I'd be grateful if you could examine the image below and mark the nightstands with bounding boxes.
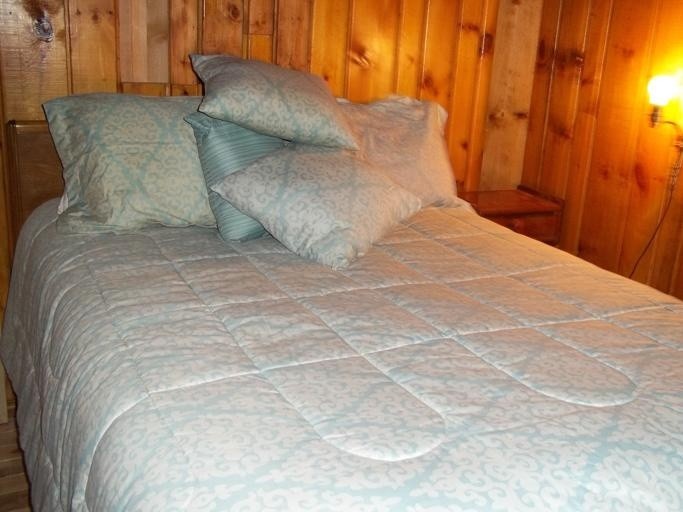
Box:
[457,184,566,250]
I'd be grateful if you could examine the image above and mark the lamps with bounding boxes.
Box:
[647,75,679,130]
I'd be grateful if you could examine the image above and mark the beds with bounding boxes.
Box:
[0,118,682,512]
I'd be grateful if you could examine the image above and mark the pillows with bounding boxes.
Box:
[188,53,362,149]
[210,143,422,271]
[338,95,462,208]
[41,91,218,234]
[183,112,292,243]
